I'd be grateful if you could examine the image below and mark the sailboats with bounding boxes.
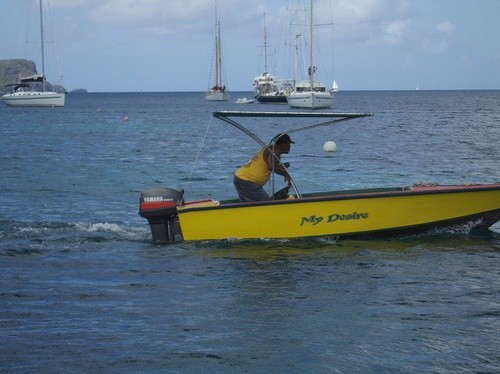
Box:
[286,0,335,108]
[331,79,339,93]
[205,0,230,100]
[253,12,294,101]
[1,0,65,106]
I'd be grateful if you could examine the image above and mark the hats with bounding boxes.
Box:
[276,133,296,144]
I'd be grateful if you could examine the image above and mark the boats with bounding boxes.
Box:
[235,98,254,104]
[139,111,500,244]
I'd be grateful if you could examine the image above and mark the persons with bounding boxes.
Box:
[233,133,296,202]
